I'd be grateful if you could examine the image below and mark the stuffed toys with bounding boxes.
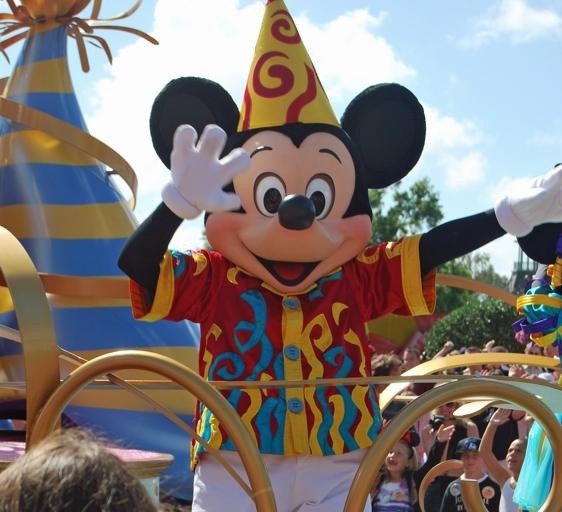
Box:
[116,74,561,512]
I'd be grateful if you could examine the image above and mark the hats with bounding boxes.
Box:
[455,438,480,454]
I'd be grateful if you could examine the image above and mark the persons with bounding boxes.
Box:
[362,339,561,512]
[0,427,155,510]
